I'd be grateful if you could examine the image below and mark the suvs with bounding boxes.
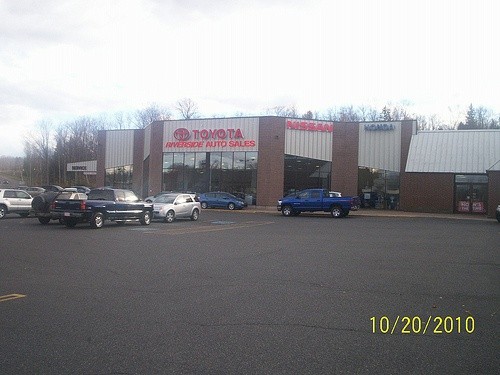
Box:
[197,190,246,210]
[32,187,91,223]
[148,192,202,222]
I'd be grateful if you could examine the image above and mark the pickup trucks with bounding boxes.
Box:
[52,188,154,228]
[276,187,362,217]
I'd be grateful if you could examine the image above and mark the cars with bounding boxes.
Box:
[25,183,63,196]
[0,188,35,218]
